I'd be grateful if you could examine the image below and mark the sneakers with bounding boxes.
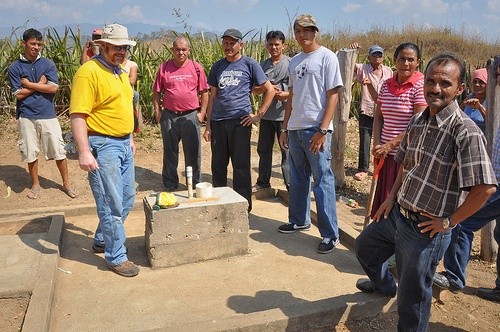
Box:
[316,237,340,254]
[92,242,104,253]
[277,222,311,234]
[107,259,140,277]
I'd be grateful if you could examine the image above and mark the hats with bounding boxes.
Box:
[221,28,243,40]
[93,24,136,46]
[294,14,319,32]
[369,45,384,53]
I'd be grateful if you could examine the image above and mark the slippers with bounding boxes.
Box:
[61,187,78,199]
[27,187,41,199]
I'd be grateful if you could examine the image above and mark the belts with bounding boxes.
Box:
[399,206,433,222]
[165,108,195,114]
[88,130,129,139]
[303,126,333,133]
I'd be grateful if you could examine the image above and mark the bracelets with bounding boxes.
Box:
[280,129,288,133]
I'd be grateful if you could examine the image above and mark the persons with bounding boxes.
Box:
[277,15,344,254]
[369,43,428,221]
[69,23,140,277]
[433,56,500,301]
[203,29,275,217]
[253,30,292,192]
[459,67,488,128]
[153,37,209,191]
[80,29,143,133]
[354,54,499,332]
[8,28,77,198]
[352,45,395,180]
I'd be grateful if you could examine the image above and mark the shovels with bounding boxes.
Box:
[357,155,386,261]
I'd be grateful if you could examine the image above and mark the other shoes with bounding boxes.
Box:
[476,286,499,301]
[159,185,178,191]
[355,278,396,298]
[354,171,368,180]
[252,183,271,193]
[432,271,461,294]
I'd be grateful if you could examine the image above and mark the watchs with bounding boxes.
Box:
[443,216,457,230]
[319,127,327,136]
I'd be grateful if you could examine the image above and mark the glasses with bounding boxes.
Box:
[371,53,383,57]
[108,44,131,50]
